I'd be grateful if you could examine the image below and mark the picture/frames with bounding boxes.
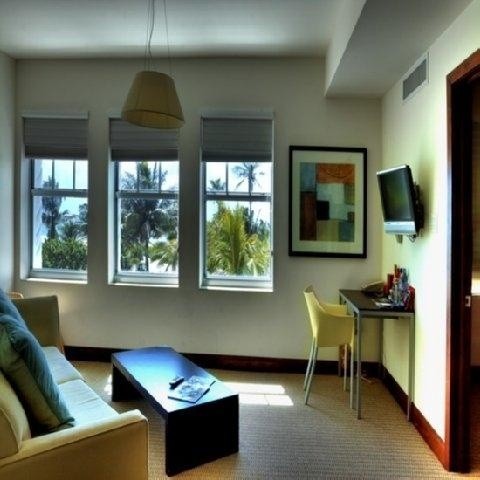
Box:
[288,145,368,259]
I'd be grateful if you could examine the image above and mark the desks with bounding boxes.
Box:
[339,289,414,421]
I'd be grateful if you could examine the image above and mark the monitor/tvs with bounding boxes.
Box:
[376,164,424,235]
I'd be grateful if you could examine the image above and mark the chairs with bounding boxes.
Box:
[303,285,355,409]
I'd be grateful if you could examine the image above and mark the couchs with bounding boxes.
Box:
[0,297,149,480]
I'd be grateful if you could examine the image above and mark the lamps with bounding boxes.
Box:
[120,0,185,129]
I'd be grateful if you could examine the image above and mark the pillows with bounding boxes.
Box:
[0,288,26,325]
[0,314,75,433]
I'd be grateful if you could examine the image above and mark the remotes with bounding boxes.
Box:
[169,375,184,386]
